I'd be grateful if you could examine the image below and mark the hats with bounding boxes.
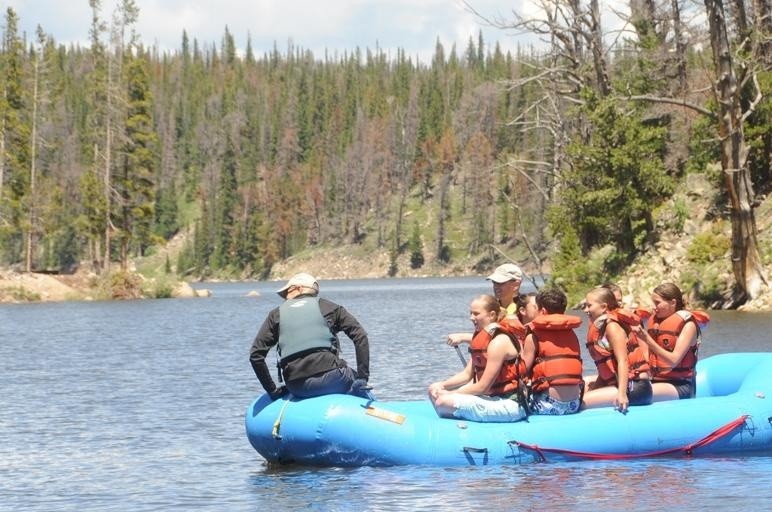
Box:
[486,263,523,285]
[276,273,320,298]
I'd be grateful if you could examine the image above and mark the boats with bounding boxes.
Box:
[244,351,771,466]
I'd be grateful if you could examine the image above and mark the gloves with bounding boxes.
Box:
[270,386,288,401]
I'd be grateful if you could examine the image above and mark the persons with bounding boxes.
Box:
[448,264,523,346]
[600,282,702,401]
[430,295,530,423]
[502,288,581,416]
[250,274,378,402]
[576,288,652,413]
